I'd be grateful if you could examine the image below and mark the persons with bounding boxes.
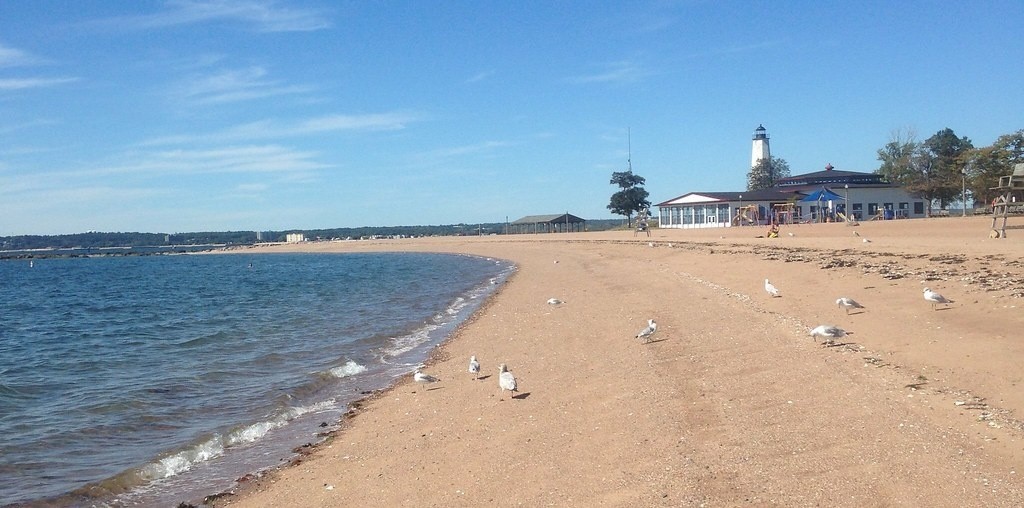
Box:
[992,198,996,211]
[836,205,844,214]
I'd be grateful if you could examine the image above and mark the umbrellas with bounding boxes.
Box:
[801,187,849,207]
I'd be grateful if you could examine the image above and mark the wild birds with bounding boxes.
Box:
[836,297,866,315]
[547,297,566,308]
[634,319,658,344]
[468,355,480,380]
[788,232,795,237]
[669,242,676,248]
[720,235,723,239]
[413,369,441,390]
[862,238,872,243]
[497,363,519,401]
[648,242,656,247]
[853,231,860,237]
[764,278,781,297]
[807,325,854,348]
[922,286,956,312]
[552,260,560,264]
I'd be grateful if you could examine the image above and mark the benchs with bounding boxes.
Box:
[938,210,950,217]
[928,211,939,218]
[1009,206,1024,213]
[973,208,989,215]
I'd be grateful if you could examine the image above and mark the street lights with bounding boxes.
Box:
[845,184,849,223]
[739,194,743,227]
[961,168,967,217]
